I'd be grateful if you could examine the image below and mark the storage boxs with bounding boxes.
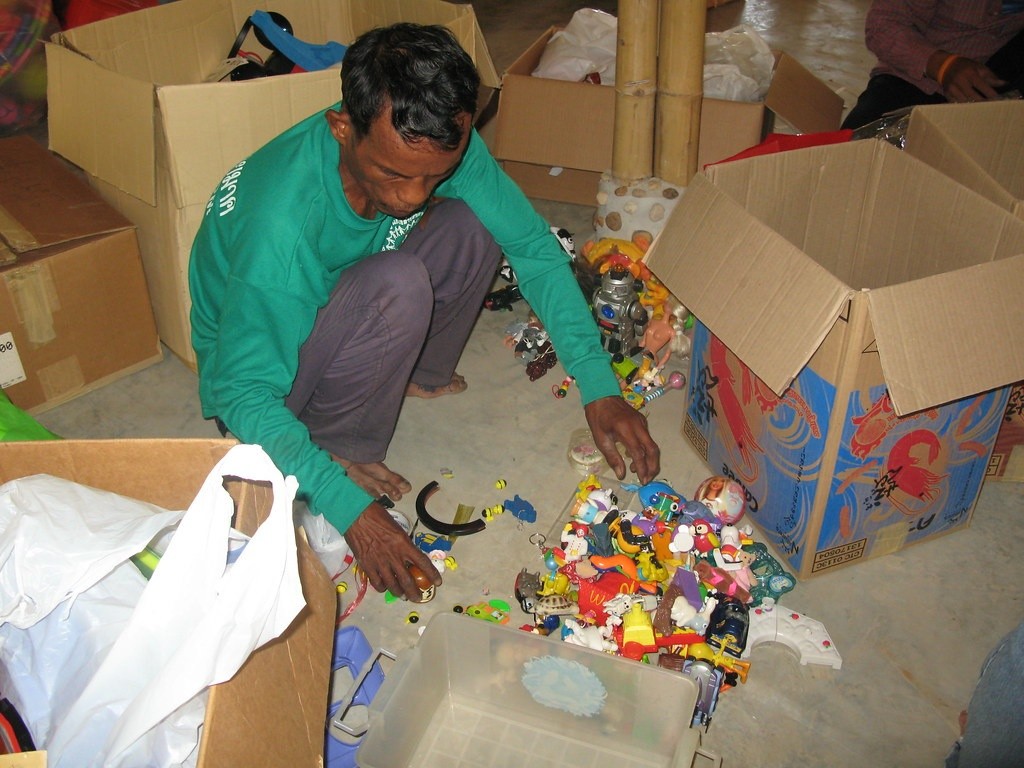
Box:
[0,437,342,768]
[326,610,721,768]
[41,2,504,377]
[900,98,1023,216]
[0,130,168,412]
[486,25,849,211]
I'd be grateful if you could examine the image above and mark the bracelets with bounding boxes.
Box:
[935,54,957,88]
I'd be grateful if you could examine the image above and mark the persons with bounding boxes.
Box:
[839,0,1024,140]
[185,22,662,605]
[616,295,692,392]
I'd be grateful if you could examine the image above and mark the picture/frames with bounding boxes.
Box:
[636,134,1024,582]
